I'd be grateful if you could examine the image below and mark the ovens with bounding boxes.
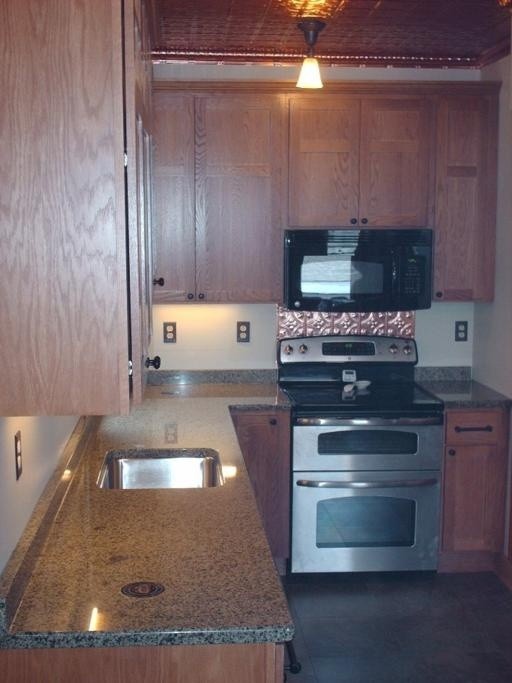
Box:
[291,408,447,575]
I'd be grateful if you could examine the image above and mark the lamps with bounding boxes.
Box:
[294,20,326,89]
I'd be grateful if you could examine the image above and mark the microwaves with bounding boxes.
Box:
[281,227,435,311]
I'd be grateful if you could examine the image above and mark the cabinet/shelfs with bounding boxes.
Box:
[445,405,502,568]
[432,89,495,301]
[231,408,291,575]
[2,0,155,416]
[152,80,286,302]
[286,86,430,227]
